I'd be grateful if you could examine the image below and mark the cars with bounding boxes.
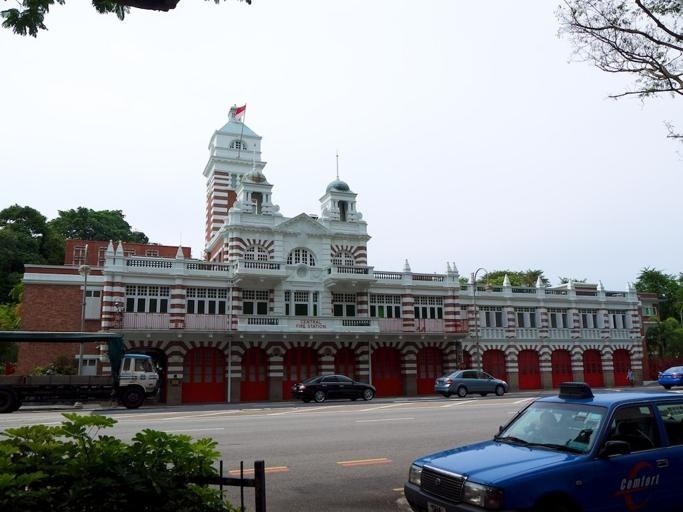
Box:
[290,374,377,403]
[656,365,683,389]
[403,381,682,512]
[434,368,508,397]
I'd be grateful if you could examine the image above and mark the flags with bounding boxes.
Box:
[234,105,246,115]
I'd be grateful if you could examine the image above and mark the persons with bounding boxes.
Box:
[625,368,635,387]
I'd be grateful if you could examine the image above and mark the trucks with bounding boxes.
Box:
[0,331,160,413]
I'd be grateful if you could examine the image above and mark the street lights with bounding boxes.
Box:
[73,244,89,408]
[226,278,243,404]
[470,268,492,369]
